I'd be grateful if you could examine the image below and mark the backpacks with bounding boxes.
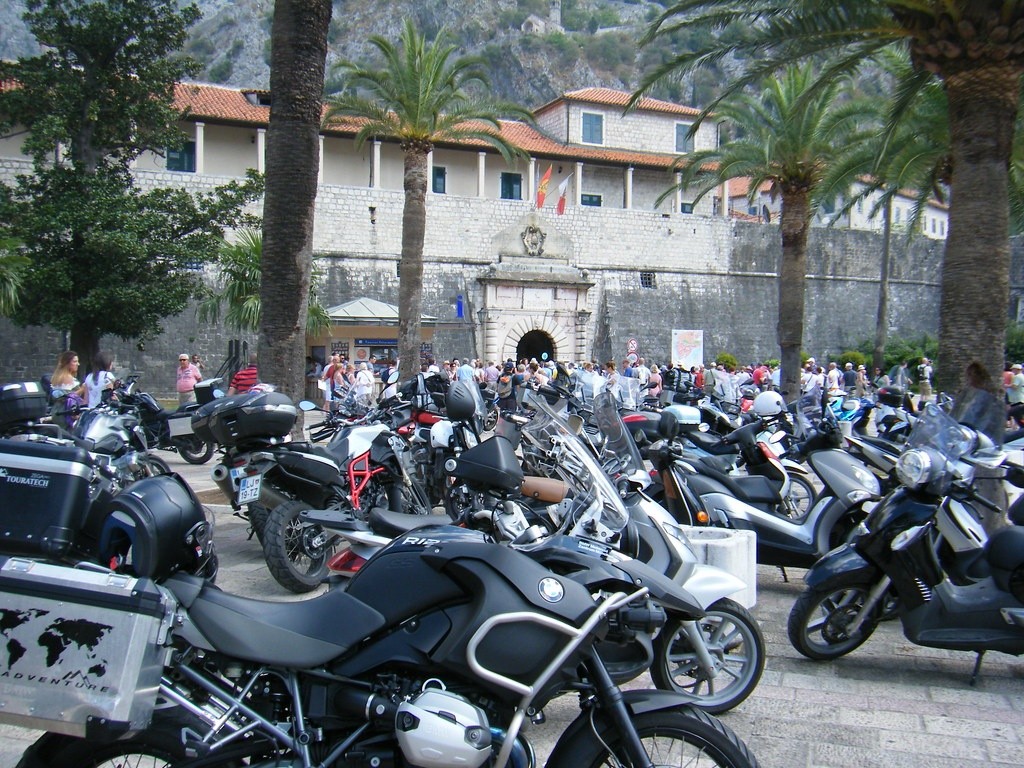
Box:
[381,367,393,382]
[917,364,927,379]
[664,369,675,386]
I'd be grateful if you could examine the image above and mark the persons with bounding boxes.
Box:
[421,354,781,410]
[1004,361,1024,429]
[50,351,85,397]
[227,353,258,395]
[177,354,203,402]
[306,352,397,417]
[84,352,116,408]
[190,354,204,370]
[918,358,934,400]
[801,357,913,394]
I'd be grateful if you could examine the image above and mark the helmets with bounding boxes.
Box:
[755,391,788,416]
[95,474,209,585]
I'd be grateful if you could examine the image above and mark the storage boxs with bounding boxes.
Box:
[192,393,298,447]
[195,377,224,404]
[878,386,903,407]
[0,378,47,428]
[661,404,700,433]
[0,438,134,561]
[624,410,661,437]
[0,555,178,745]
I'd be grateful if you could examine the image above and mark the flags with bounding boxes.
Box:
[537,166,552,208]
[557,178,568,216]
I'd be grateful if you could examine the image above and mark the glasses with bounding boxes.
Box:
[179,359,187,361]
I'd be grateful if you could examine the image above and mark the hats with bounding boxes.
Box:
[548,361,555,369]
[710,362,717,366]
[463,358,469,364]
[845,363,852,368]
[746,365,753,370]
[506,362,514,369]
[530,358,538,364]
[1010,364,1022,369]
[857,365,865,370]
[807,357,815,363]
[443,360,450,364]
[717,365,724,370]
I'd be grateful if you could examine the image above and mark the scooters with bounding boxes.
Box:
[786,404,1023,687]
[0,336,1024,716]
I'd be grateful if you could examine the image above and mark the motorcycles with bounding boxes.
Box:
[1,376,761,768]
